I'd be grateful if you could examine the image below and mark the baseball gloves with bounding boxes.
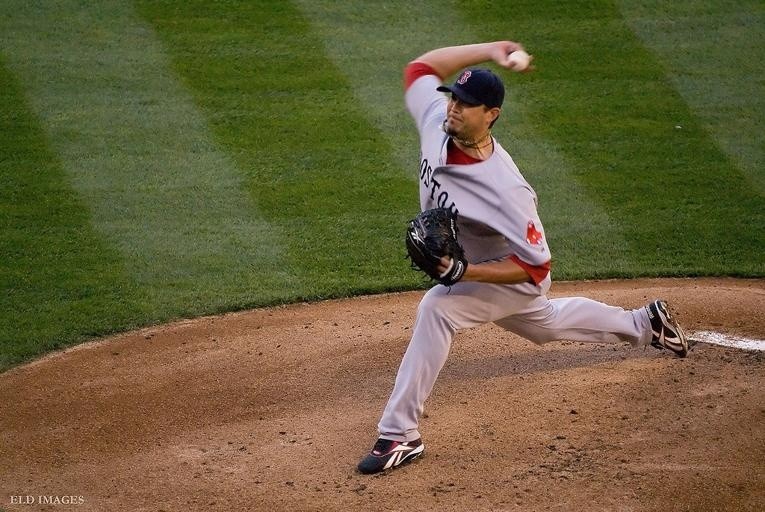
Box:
[406,207,468,289]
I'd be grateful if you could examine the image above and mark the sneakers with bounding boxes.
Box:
[358,438,424,475]
[645,298,688,357]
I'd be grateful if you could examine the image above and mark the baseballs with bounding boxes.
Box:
[508,51,530,73]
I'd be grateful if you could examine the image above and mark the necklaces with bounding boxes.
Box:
[465,140,493,149]
[443,118,490,146]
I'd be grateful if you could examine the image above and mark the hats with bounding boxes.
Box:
[436,68,504,108]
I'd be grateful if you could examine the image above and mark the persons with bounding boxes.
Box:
[354,39,691,474]
